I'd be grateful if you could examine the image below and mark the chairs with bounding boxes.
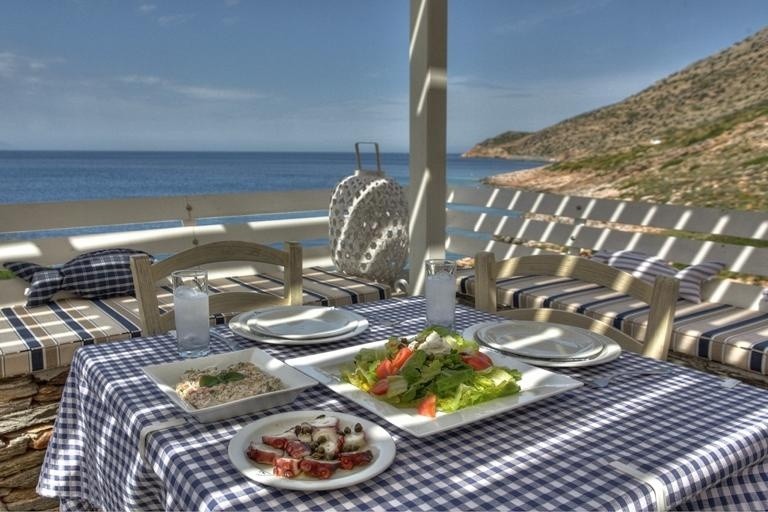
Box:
[129,239,307,337]
[472,250,679,360]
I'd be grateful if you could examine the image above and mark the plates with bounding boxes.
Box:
[229,309,370,345]
[464,323,622,368]
[139,346,320,423]
[287,335,584,441]
[226,410,397,494]
[247,306,357,337]
[478,322,603,361]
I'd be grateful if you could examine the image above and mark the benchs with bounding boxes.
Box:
[1,267,393,379]
[458,259,768,380]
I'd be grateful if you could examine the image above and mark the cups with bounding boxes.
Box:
[171,269,210,358]
[423,259,457,332]
